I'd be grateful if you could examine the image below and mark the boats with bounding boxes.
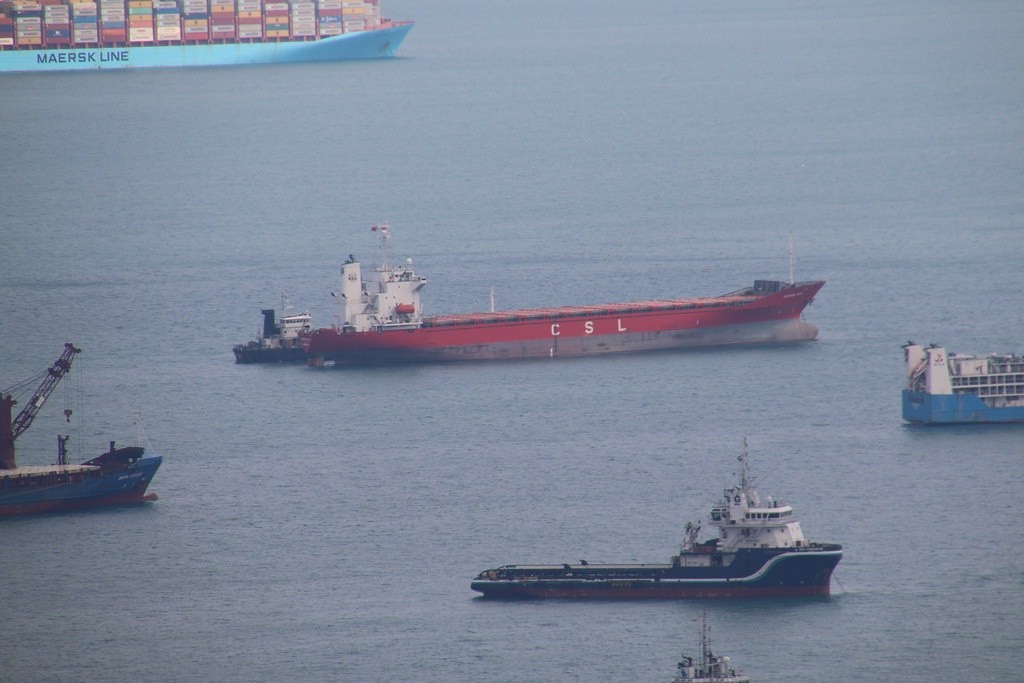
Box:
[469,435,843,599]
[901,341,1024,425]
[233,293,333,364]
[673,609,751,683]
[298,223,830,366]
[0,0,416,72]
[0,342,165,516]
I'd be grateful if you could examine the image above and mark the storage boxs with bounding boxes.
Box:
[0,0,381,50]
